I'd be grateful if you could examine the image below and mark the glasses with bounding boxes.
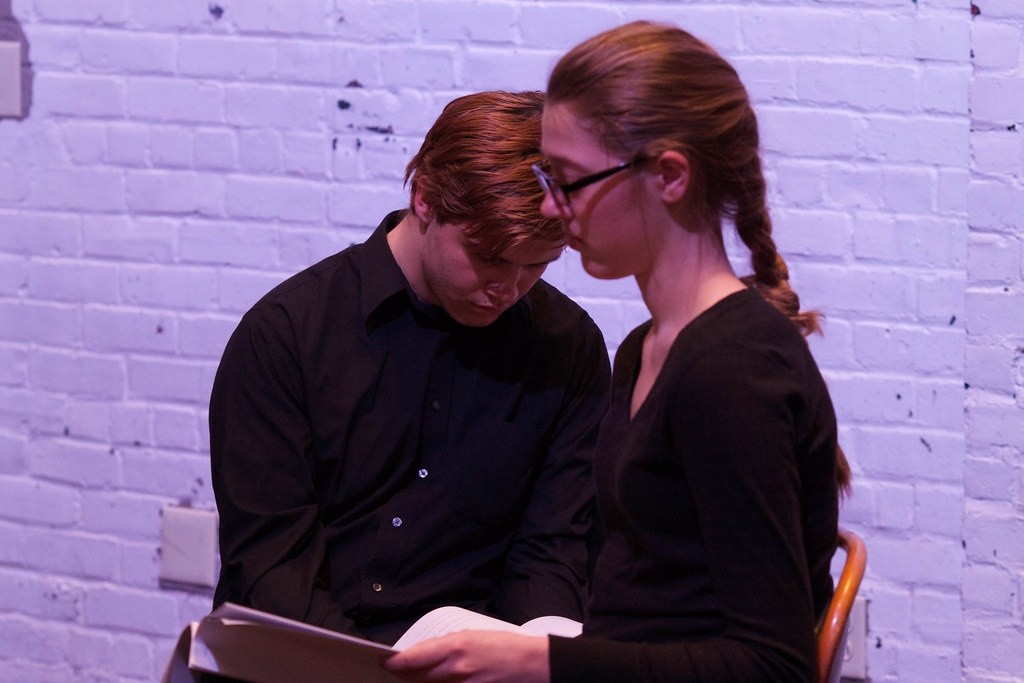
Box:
[532,159,641,206]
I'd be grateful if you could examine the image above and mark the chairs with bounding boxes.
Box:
[815,526,866,683]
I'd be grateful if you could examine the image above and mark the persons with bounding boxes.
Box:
[206,90,611,645]
[387,21,852,683]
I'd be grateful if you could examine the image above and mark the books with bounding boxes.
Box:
[162,602,584,683]
